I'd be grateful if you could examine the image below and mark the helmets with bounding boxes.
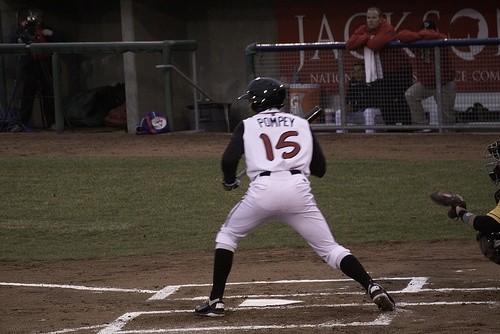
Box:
[483,141,500,183]
[237,77,287,107]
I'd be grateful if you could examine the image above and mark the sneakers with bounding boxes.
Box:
[194,298,225,318]
[367,280,396,312]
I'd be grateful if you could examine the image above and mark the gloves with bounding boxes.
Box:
[222,179,241,191]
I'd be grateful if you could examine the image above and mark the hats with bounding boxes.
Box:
[420,19,436,30]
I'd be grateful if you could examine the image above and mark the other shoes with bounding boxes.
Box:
[423,128,439,133]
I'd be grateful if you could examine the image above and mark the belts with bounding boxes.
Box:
[260,169,301,177]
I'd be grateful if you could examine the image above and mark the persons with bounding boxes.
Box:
[432,140,500,265]
[396,21,462,132]
[10,9,54,131]
[343,8,415,130]
[195,77,395,317]
[347,63,369,112]
